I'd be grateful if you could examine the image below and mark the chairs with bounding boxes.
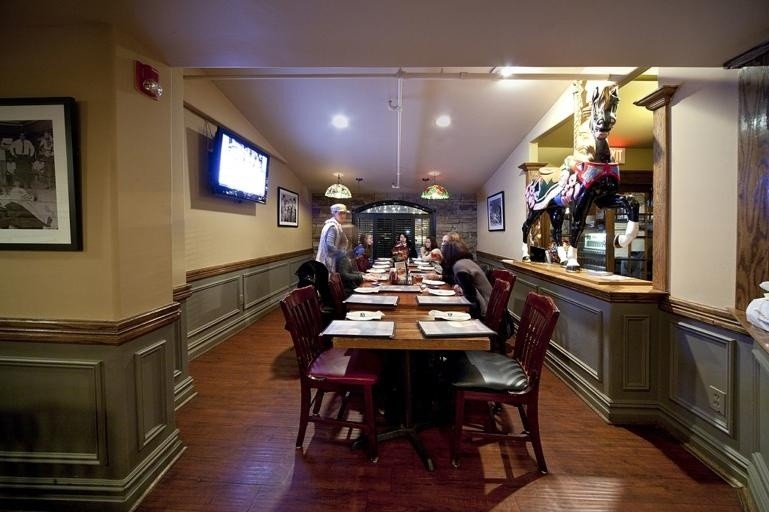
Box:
[293,254,517,410]
[434,292,560,476]
[279,283,383,464]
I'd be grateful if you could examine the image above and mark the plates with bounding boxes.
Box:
[363,257,391,281]
[432,289,455,295]
[357,287,374,293]
[426,280,446,284]
[441,311,471,321]
[349,310,374,320]
[412,257,434,270]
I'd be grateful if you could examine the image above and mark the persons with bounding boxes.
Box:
[336,231,514,346]
[280,195,296,223]
[0,129,55,192]
[315,203,352,281]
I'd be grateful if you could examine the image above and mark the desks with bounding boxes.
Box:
[333,311,494,473]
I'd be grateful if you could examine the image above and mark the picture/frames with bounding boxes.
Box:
[276,185,300,229]
[486,191,506,232]
[1,94,85,254]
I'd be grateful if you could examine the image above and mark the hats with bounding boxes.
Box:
[330,204,350,214]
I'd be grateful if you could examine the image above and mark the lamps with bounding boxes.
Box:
[421,169,450,201]
[134,60,163,102]
[322,169,352,200]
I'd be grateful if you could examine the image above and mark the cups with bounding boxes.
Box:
[420,283,427,292]
[415,274,423,285]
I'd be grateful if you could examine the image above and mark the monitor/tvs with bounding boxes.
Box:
[208,125,271,205]
